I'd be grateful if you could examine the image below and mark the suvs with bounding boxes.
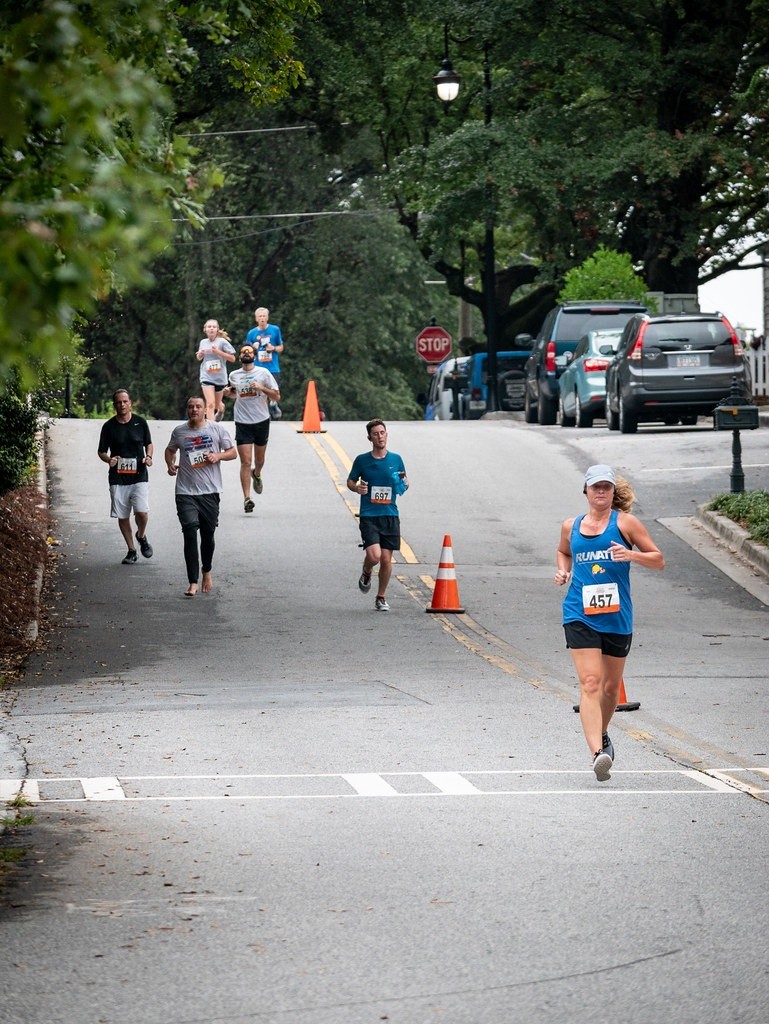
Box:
[514,299,651,428]
[600,311,754,435]
[449,349,538,419]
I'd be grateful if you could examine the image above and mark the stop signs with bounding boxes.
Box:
[415,327,453,364]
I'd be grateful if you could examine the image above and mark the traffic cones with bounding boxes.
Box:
[573,666,641,712]
[296,379,329,434]
[423,534,469,613]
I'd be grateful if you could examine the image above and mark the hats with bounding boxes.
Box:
[583,465,617,487]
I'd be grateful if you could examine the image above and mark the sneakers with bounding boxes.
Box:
[376,595,390,611]
[602,733,615,761]
[122,550,138,565]
[136,531,153,558]
[593,749,613,781]
[244,499,255,513]
[251,469,263,493]
[359,571,371,594]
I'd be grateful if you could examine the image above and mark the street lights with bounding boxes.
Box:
[433,12,501,422]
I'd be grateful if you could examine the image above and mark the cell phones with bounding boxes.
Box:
[361,480,367,487]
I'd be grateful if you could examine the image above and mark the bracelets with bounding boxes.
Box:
[274,346,276,351]
[146,455,152,459]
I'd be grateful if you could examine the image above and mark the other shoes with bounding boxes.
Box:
[271,403,282,419]
[215,405,225,422]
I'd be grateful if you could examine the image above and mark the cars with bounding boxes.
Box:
[553,328,626,425]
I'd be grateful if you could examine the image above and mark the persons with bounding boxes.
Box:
[196,320,237,422]
[222,344,280,513]
[555,464,665,781]
[246,307,284,418]
[165,395,237,597]
[98,389,153,565]
[347,419,409,611]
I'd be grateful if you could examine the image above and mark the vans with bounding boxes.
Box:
[418,354,471,419]
